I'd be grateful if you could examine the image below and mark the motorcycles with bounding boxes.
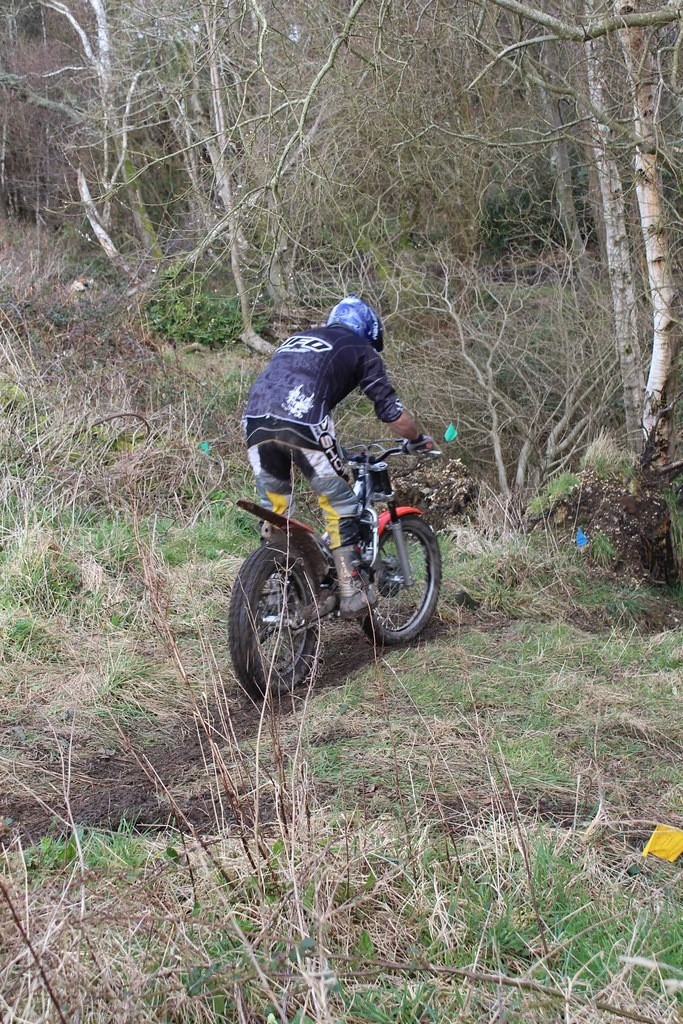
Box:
[218,441,446,703]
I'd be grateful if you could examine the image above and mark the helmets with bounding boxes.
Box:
[327,296,384,352]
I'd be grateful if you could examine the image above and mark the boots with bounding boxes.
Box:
[331,544,378,618]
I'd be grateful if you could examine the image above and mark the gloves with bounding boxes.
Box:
[411,434,433,453]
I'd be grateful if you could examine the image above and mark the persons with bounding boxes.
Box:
[241,298,435,619]
